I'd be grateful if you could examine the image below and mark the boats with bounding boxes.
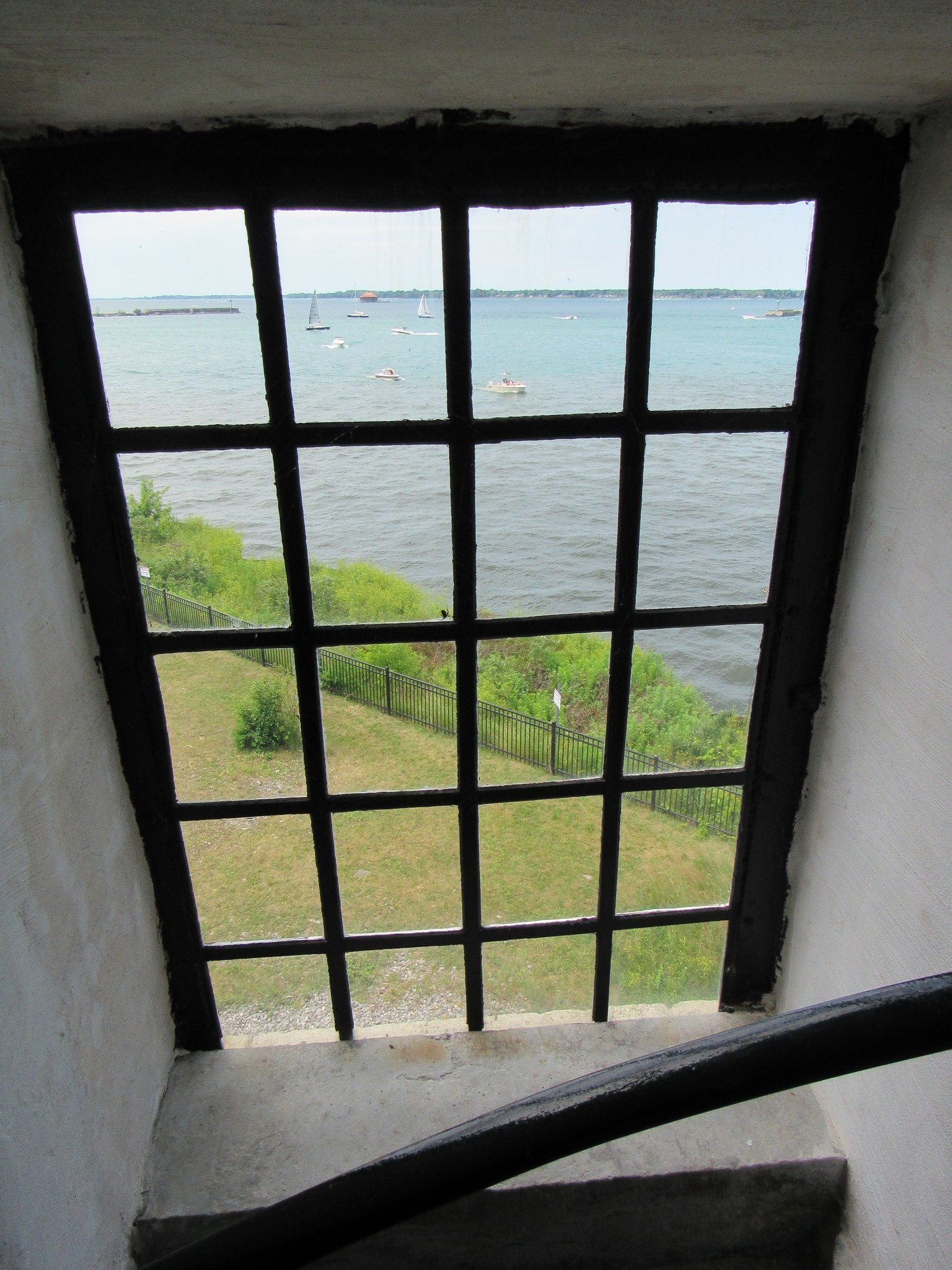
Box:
[346,282,370,318]
[320,336,350,351]
[390,327,414,335]
[730,307,735,310]
[485,373,526,392]
[741,315,755,318]
[567,315,577,319]
[374,369,399,380]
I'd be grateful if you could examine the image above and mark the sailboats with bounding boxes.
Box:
[416,293,436,318]
[305,289,330,330]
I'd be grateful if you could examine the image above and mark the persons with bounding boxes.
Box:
[389,370,393,375]
